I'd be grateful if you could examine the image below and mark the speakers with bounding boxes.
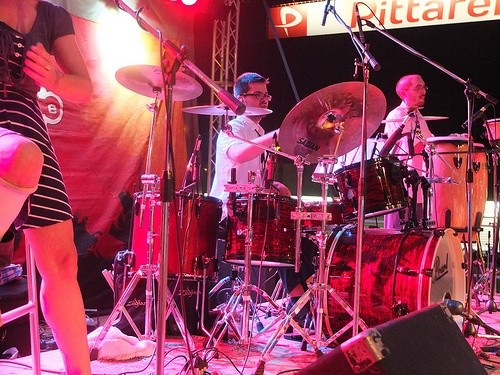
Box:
[293,301,489,375]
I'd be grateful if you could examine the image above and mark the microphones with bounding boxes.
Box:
[271,132,278,163]
[444,299,477,322]
[321,0,330,26]
[379,112,413,157]
[184,135,201,184]
[355,4,366,43]
[461,104,490,129]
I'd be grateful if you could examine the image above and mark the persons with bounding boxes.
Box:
[384,75,433,231]
[0,0,92,375]
[209,72,319,330]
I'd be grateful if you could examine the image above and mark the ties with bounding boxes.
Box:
[255,129,265,163]
[408,111,421,139]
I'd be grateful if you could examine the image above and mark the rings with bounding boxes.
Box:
[45,64,51,70]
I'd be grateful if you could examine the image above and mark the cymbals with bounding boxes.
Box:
[115,65,204,102]
[381,115,447,123]
[180,103,273,116]
[277,81,386,163]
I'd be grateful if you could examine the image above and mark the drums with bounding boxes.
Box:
[484,117,500,151]
[221,193,303,267]
[311,221,465,346]
[300,201,344,230]
[333,157,409,222]
[127,190,221,280]
[427,135,488,243]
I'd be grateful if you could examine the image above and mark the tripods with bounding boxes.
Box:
[476,209,500,315]
[90,0,381,375]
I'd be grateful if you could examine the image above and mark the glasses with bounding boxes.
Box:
[240,91,272,101]
[407,86,428,92]
